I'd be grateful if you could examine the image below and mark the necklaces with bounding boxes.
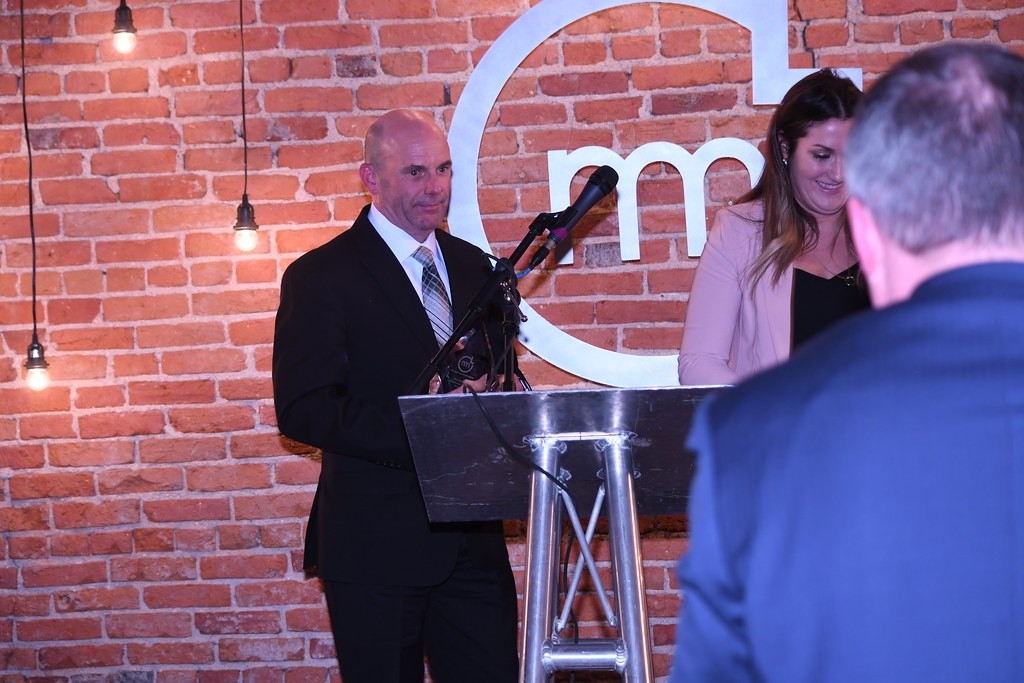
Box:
[806,231,856,287]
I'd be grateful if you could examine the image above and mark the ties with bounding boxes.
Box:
[410,246,454,350]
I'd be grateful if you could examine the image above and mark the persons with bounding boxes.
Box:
[674,38,1024,683]
[272,108,520,683]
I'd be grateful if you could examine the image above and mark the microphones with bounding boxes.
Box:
[531,164,619,268]
